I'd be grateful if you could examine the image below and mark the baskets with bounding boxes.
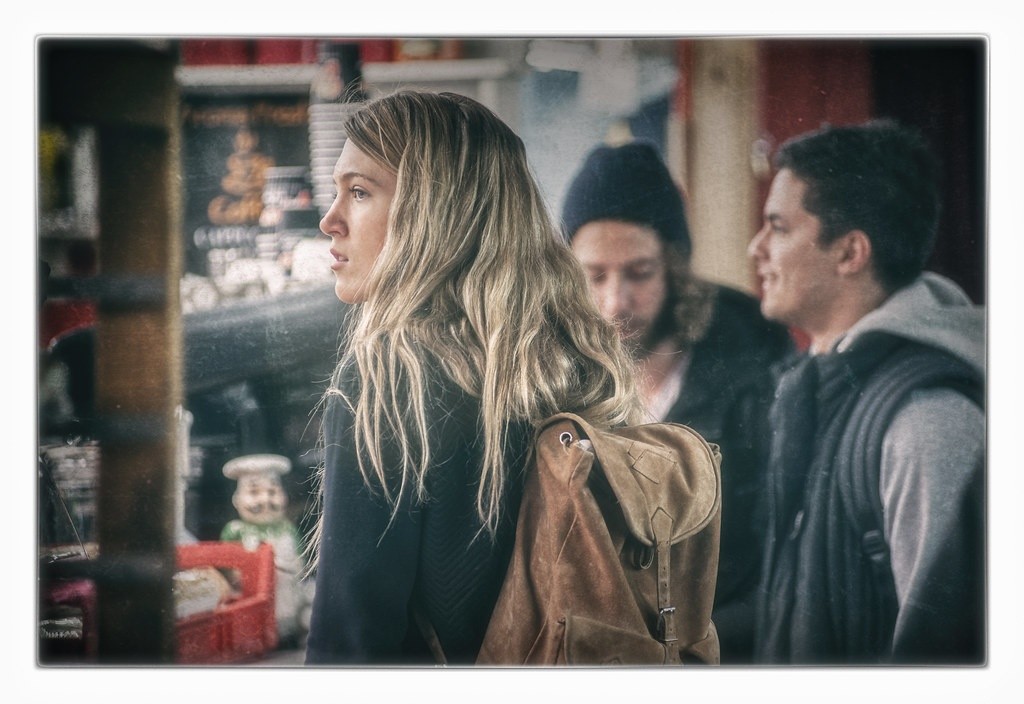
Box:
[172,541,276,663]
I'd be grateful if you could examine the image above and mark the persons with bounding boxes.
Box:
[214,456,308,644]
[300,90,653,666]
[747,118,984,665]
[554,139,796,664]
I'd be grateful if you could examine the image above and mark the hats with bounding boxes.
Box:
[557,138,693,263]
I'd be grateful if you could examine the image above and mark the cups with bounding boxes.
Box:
[311,43,365,207]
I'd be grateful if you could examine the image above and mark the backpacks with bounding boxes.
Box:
[473,411,722,667]
